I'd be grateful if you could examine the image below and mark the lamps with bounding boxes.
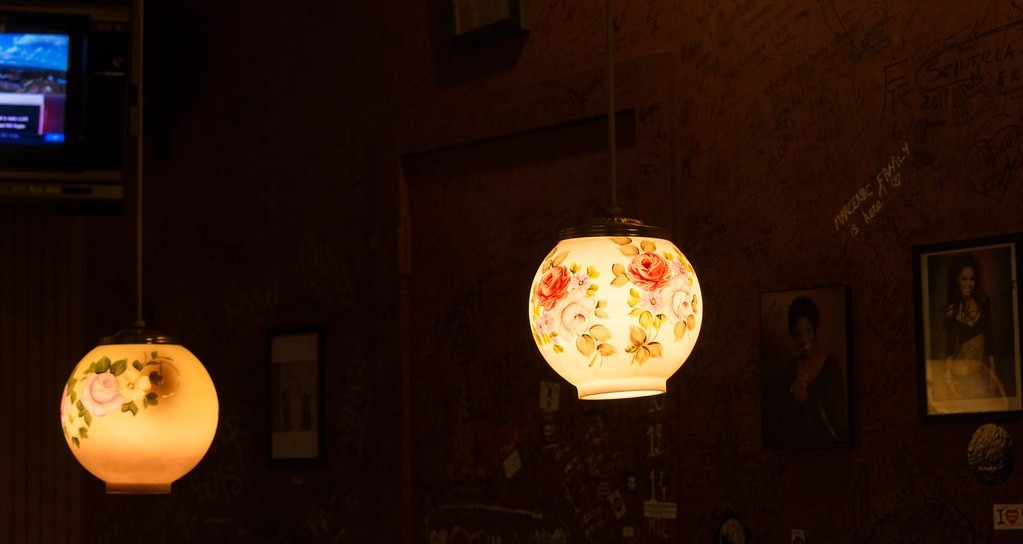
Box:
[526,0,703,400]
[59,0,224,495]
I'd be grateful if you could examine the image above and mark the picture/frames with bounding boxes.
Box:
[912,230,1023,424]
[758,283,856,450]
[264,322,327,467]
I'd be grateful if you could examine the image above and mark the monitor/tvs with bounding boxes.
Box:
[0,10,91,172]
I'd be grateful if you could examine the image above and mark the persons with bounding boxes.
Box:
[929,258,1005,401]
[764,294,848,445]
